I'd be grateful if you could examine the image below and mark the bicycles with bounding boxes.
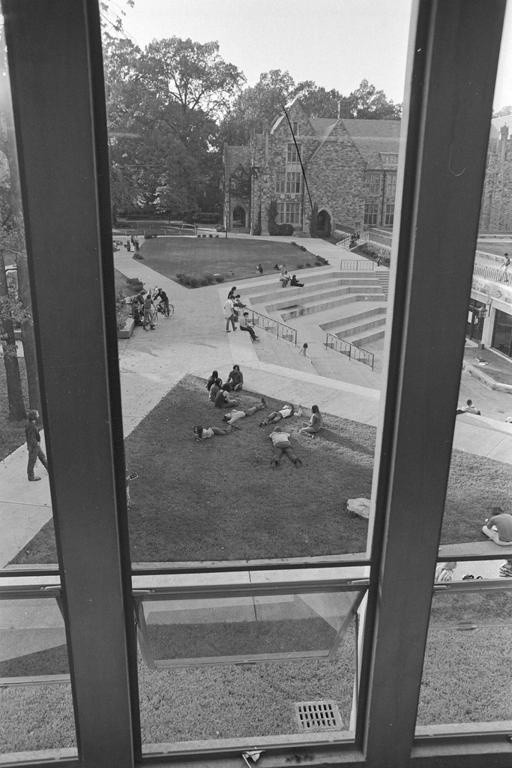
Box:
[133,300,174,331]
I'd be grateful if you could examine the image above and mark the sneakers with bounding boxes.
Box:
[29,476,41,481]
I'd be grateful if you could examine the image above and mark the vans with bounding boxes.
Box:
[196,228,218,238]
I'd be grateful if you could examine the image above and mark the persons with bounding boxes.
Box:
[131,288,169,331]
[481,506,512,546]
[24,410,48,482]
[205,364,243,411]
[498,558,512,577]
[435,561,457,583]
[256,263,263,275]
[463,399,481,416]
[124,234,140,252]
[275,263,304,288]
[299,343,308,357]
[268,426,303,474]
[298,403,323,439]
[495,251,511,283]
[221,396,267,425]
[223,285,258,342]
[259,402,295,428]
[193,423,242,443]
